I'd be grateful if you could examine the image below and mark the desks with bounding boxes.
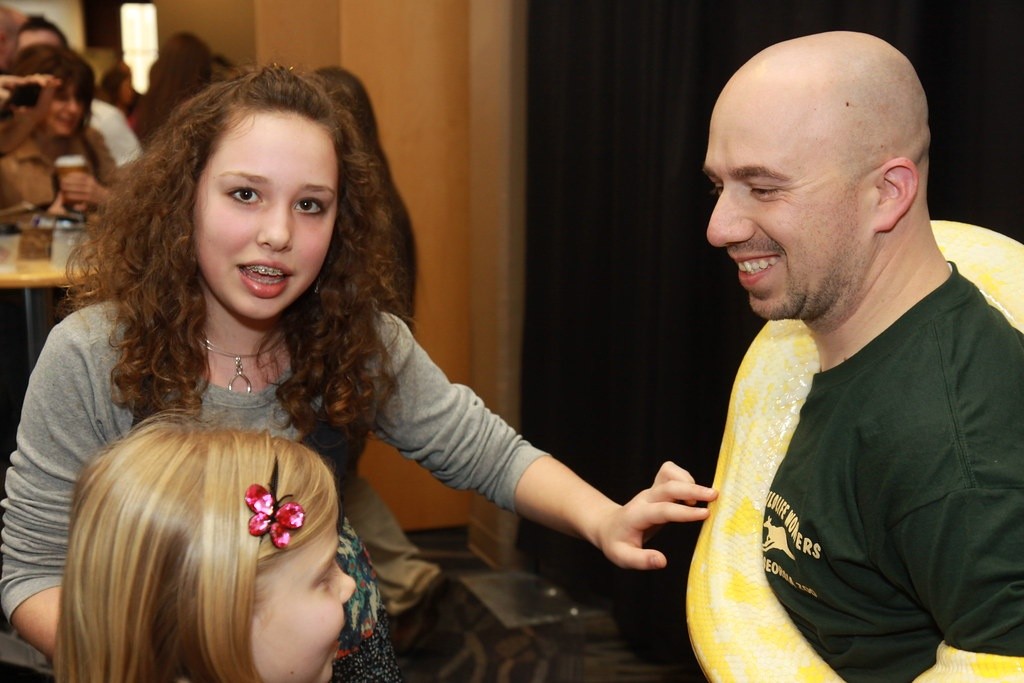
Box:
[0,230,99,370]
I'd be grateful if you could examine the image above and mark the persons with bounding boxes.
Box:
[0,1,238,452]
[1,65,719,683]
[52,407,357,683]
[703,30,1024,683]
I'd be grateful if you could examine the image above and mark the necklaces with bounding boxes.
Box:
[202,341,283,393]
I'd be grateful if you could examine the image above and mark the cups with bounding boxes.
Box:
[53,154,89,203]
